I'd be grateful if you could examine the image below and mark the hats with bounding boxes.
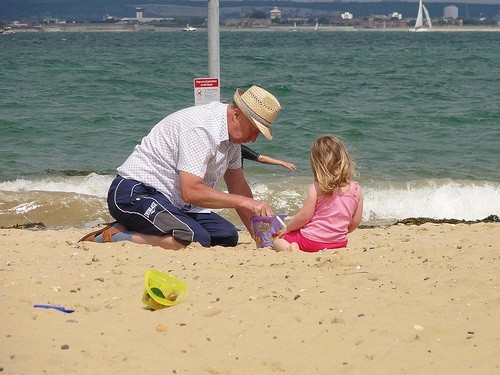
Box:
[233,85,281,141]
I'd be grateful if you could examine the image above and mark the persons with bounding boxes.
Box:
[76,85,282,250]
[241,142,297,172]
[272,134,363,253]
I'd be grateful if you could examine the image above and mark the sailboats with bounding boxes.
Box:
[407,0,433,33]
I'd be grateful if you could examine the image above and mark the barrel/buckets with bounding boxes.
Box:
[249,215,288,249]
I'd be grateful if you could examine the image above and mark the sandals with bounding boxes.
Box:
[78,219,128,242]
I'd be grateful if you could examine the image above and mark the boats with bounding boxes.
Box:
[3,27,16,35]
[182,24,197,31]
